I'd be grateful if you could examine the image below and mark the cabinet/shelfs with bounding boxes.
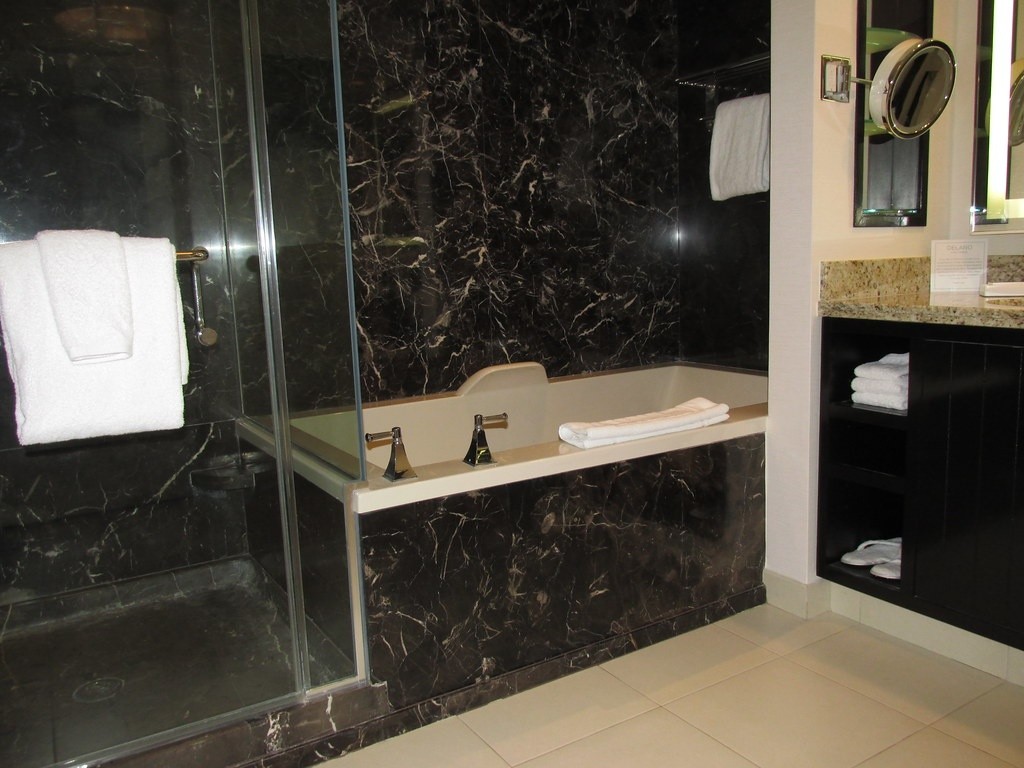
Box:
[815,317,1023,652]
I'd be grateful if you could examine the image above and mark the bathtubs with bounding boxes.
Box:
[234,353,771,709]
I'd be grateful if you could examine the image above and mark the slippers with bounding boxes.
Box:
[870,559,901,579]
[841,537,901,565]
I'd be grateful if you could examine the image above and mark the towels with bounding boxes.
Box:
[708,91,770,203]
[0,232,190,448]
[850,351,911,411]
[556,397,729,451]
[35,226,133,361]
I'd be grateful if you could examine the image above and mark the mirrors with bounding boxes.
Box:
[822,36,956,140]
[977,58,1024,146]
[855,0,934,227]
[969,0,1024,235]
